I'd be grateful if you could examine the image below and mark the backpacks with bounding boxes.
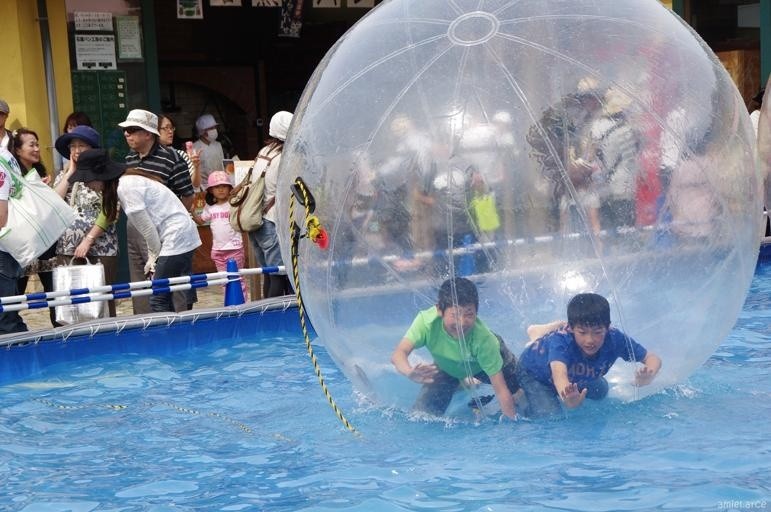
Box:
[227,145,280,234]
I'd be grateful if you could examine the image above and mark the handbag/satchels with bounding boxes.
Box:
[0,153,80,269]
[49,254,107,327]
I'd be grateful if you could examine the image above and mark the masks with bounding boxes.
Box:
[201,129,219,144]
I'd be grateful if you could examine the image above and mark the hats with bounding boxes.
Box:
[201,169,233,194]
[193,112,221,136]
[53,124,104,164]
[116,108,162,137]
[566,74,638,118]
[0,98,8,115]
[64,148,126,185]
[266,108,294,144]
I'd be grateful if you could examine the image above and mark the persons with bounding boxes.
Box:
[350,112,522,274]
[515,292,664,421]
[389,277,520,422]
[558,74,762,257]
[0,100,317,335]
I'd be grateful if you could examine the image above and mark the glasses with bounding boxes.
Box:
[119,126,146,135]
[158,124,176,133]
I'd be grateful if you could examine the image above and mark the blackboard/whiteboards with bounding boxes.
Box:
[71,70,131,163]
